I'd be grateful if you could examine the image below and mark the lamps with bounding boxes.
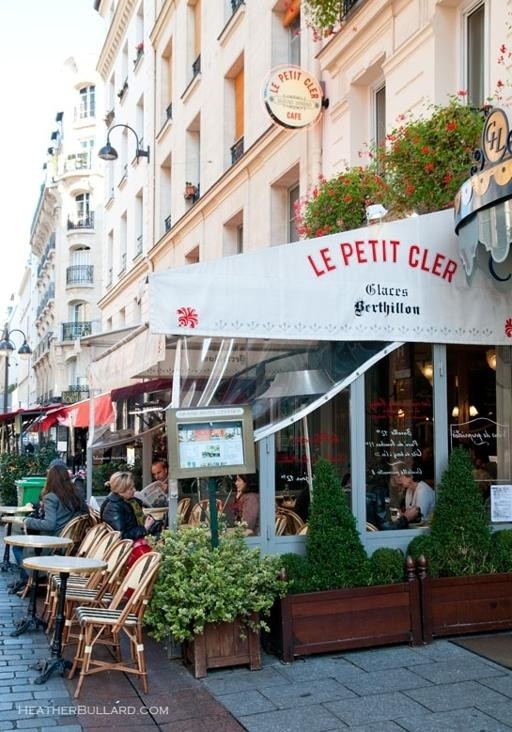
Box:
[98,124,149,164]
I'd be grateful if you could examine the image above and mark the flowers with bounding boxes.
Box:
[284,0,358,43]
[286,90,494,245]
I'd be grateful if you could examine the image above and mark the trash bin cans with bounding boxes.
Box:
[13,476,49,509]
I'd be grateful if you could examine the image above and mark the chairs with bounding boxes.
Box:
[176,498,378,535]
[19,503,164,696]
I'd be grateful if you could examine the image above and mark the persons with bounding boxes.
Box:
[151,461,185,505]
[232,468,259,538]
[11,464,89,597]
[339,451,495,532]
[26,458,85,521]
[99,471,156,541]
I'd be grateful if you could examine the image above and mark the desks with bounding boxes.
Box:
[0,506,37,575]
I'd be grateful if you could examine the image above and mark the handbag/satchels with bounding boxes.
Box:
[21,513,43,536]
[148,514,164,539]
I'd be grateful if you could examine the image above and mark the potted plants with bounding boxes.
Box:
[261,443,512,661]
[186,181,196,195]
[142,517,292,678]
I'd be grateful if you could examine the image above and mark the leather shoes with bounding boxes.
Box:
[16,586,32,597]
